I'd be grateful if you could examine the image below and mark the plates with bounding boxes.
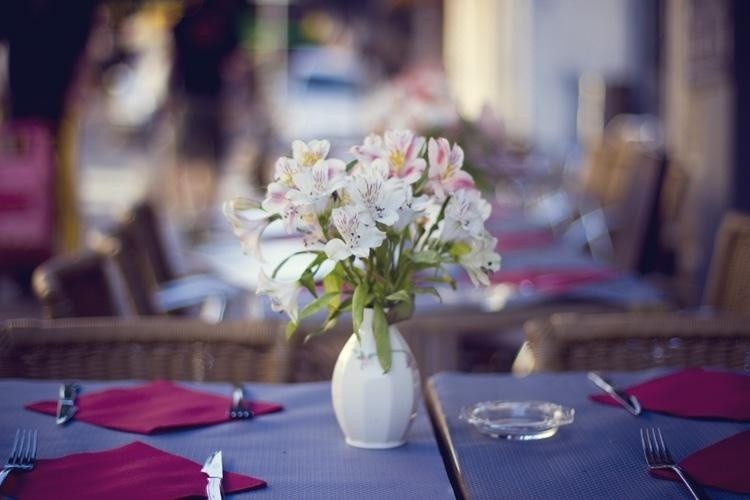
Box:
[460,402,575,440]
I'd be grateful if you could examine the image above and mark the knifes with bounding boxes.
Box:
[56,383,82,427]
[587,369,643,415]
[200,449,226,499]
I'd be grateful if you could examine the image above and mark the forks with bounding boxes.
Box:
[640,428,707,500]
[226,385,254,421]
[1,427,38,499]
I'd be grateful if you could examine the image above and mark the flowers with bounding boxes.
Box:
[222,126,502,373]
[367,61,526,202]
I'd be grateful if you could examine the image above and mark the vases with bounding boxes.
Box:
[331,307,422,449]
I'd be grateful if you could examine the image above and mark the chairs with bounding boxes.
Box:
[3,197,297,381]
[511,131,750,374]
